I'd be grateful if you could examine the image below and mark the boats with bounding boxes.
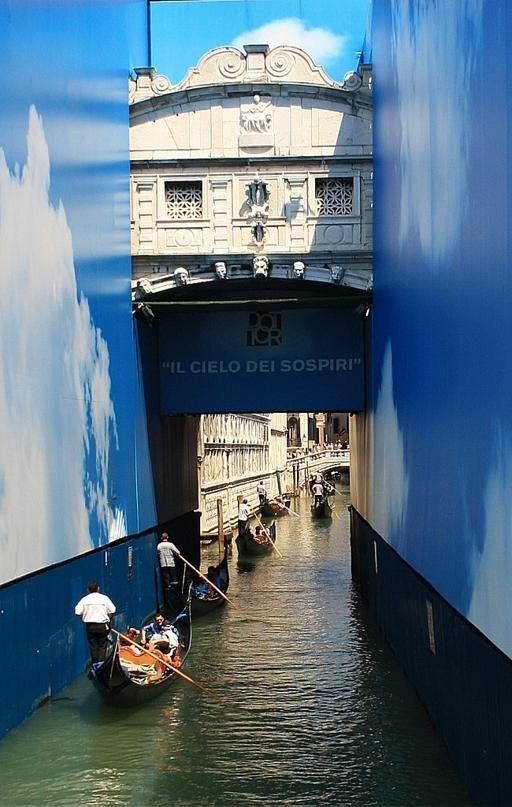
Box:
[166,535,229,614]
[259,496,290,517]
[234,514,276,554]
[309,481,335,518]
[92,581,192,708]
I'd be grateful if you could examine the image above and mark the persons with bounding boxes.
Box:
[238,499,250,535]
[253,470,334,542]
[213,568,224,589]
[141,613,179,645]
[75,581,116,669]
[161,620,179,656]
[203,566,215,583]
[157,533,180,593]
[287,439,347,466]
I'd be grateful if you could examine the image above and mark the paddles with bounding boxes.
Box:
[322,494,341,521]
[323,478,342,496]
[111,627,226,702]
[175,551,241,611]
[251,509,282,559]
[267,493,300,518]
